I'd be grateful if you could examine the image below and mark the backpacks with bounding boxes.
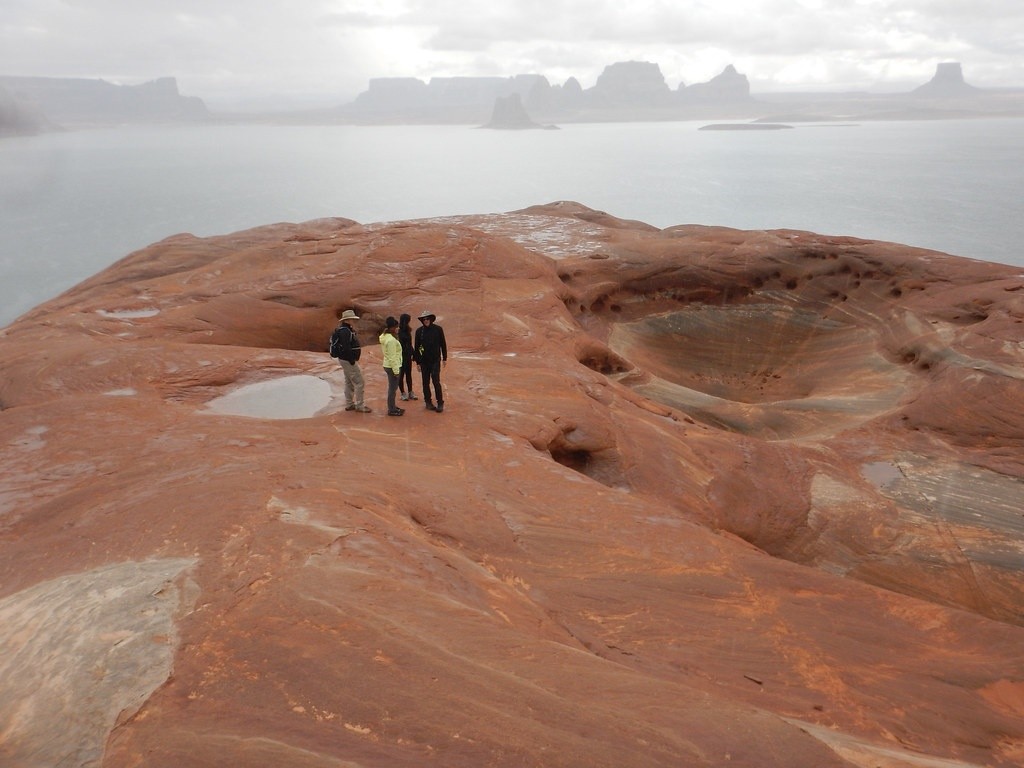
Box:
[329,326,356,358]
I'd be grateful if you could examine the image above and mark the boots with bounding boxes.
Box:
[424,399,436,410]
[436,400,444,412]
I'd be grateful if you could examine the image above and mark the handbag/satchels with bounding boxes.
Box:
[418,326,425,356]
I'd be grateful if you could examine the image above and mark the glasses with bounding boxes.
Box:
[422,318,429,320]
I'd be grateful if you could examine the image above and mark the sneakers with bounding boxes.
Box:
[408,391,418,400]
[344,401,357,411]
[354,401,372,413]
[400,392,408,401]
[387,406,405,416]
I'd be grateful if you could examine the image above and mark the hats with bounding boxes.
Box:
[418,310,436,323]
[386,316,398,328]
[339,309,359,321]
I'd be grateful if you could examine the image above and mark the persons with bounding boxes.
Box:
[398,313,418,401]
[335,310,371,413]
[415,311,448,412]
[379,317,405,416]
